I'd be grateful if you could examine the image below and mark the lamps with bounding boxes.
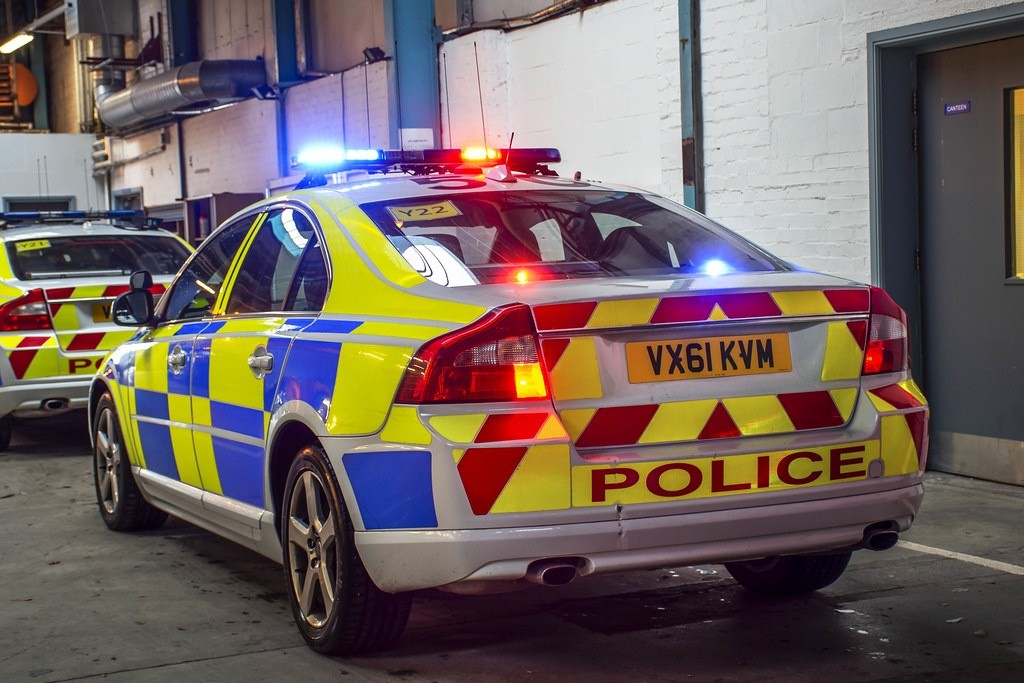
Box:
[364,47,385,62]
[0,31,36,54]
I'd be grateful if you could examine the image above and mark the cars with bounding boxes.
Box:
[1,211,223,442]
[85,149,929,652]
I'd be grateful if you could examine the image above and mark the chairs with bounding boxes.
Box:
[414,224,691,271]
[111,240,168,275]
